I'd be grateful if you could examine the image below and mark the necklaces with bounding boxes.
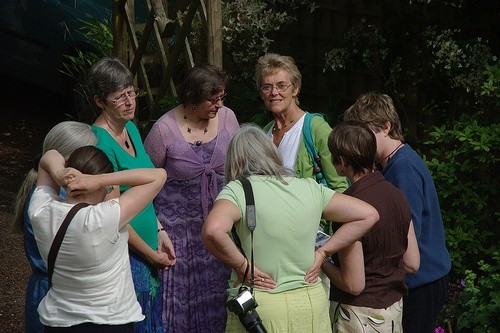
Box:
[376,142,404,165]
[103,115,130,149]
[273,120,294,132]
[183,106,208,146]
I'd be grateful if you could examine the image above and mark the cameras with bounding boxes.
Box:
[226,287,267,333]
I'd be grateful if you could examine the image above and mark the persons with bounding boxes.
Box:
[12,53,452,333]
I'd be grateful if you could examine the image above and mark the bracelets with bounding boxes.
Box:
[158,228,164,231]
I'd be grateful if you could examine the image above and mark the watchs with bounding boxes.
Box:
[233,256,246,272]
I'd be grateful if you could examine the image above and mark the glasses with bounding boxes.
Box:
[104,86,140,105]
[259,82,295,93]
[202,90,228,105]
